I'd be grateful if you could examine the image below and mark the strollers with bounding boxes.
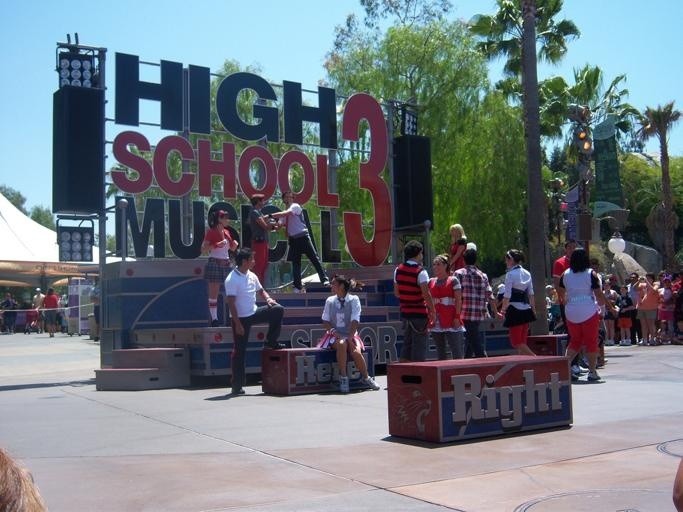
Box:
[24,307,40,334]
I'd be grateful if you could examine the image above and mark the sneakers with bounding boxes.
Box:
[263,339,285,349]
[361,375,379,390]
[338,374,349,393]
[570,356,608,381]
[605,337,656,346]
[232,387,246,396]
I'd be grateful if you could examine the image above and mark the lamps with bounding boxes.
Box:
[55,45,95,88]
[56,218,94,262]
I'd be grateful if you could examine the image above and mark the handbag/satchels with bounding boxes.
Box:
[317,327,336,349]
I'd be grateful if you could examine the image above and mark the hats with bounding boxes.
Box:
[497,284,506,294]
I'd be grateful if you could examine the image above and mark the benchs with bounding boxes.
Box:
[260,344,375,397]
[525,332,605,369]
[384,354,574,444]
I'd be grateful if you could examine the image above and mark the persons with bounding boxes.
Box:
[224,247,286,395]
[317,274,380,394]
[199,208,241,326]
[1,280,100,342]
[260,189,333,293]
[545,239,682,384]
[393,224,537,362]
[244,192,279,290]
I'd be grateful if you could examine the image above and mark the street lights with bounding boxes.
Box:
[58,50,94,88]
[573,127,626,268]
[58,226,93,262]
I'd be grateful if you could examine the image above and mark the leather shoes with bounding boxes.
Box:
[210,317,218,326]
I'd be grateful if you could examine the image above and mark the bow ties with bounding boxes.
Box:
[338,299,345,310]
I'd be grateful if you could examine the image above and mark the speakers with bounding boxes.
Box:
[53,84,103,214]
[394,134,433,233]
